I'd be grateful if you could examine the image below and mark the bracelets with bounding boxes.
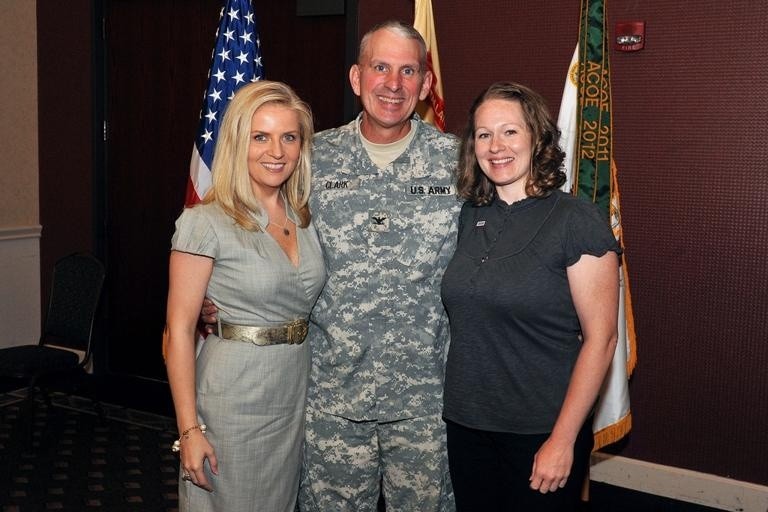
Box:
[171,423,207,453]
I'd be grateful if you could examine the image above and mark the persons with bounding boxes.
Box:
[201,19,468,512]
[440,80,622,512]
[161,78,327,512]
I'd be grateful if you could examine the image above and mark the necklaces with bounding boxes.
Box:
[269,217,290,237]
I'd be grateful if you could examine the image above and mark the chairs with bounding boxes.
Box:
[0,250,106,454]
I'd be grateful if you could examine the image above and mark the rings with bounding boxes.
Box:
[181,473,189,481]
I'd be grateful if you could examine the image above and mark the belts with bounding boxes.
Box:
[212,318,309,347]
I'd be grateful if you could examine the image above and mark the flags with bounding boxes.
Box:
[412,0,445,132]
[183,0,264,211]
[554,0,638,454]
